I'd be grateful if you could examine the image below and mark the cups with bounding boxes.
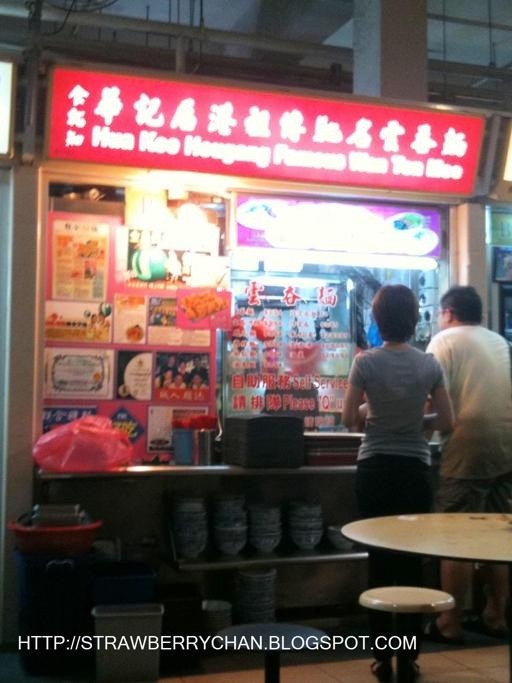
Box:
[172,427,217,466]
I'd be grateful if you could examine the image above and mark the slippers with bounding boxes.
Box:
[461,614,509,640]
[421,618,465,645]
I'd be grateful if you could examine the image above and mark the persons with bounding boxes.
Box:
[153,353,209,390]
[342,283,454,683]
[424,284,511,645]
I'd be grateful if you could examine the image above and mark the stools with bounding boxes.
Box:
[215,624,332,683]
[359,585,453,681]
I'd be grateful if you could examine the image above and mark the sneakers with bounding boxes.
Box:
[371,660,392,682]
[398,661,419,682]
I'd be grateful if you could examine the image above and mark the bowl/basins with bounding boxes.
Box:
[174,493,357,560]
[7,518,104,561]
[241,566,278,625]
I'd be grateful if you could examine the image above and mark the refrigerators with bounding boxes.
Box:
[486,244,512,346]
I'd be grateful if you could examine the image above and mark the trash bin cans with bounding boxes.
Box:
[91,603,164,683]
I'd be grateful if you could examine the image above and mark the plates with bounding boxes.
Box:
[202,599,233,634]
